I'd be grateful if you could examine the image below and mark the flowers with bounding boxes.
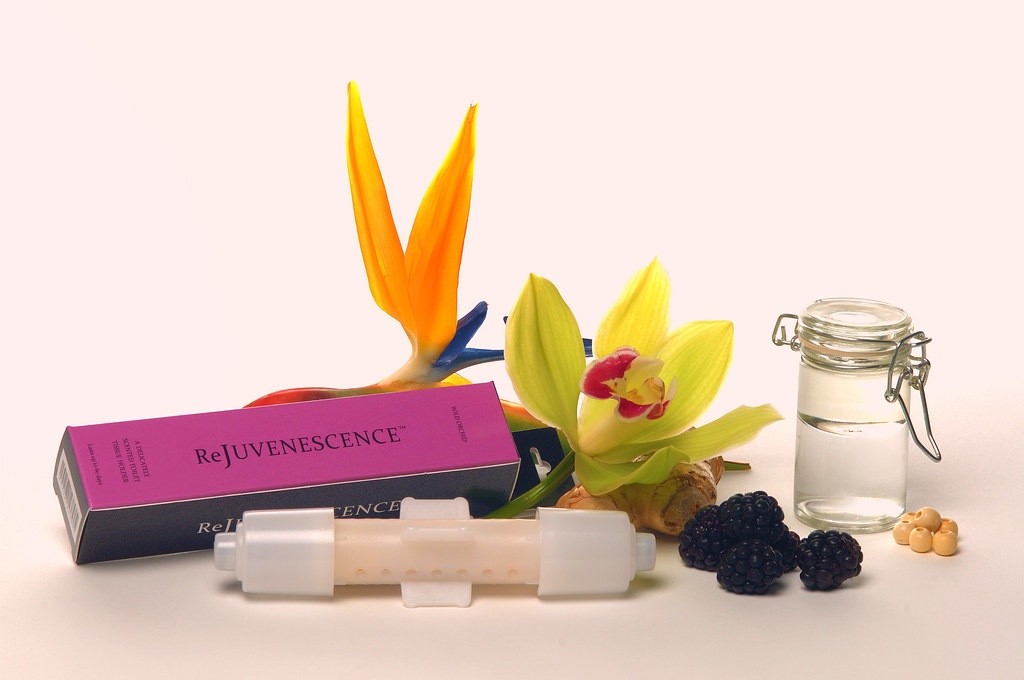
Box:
[473,254,783,520]
[244,81,606,433]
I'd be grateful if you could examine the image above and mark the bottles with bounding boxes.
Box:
[772,299,941,537]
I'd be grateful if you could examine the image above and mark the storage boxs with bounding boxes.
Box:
[53,380,523,566]
